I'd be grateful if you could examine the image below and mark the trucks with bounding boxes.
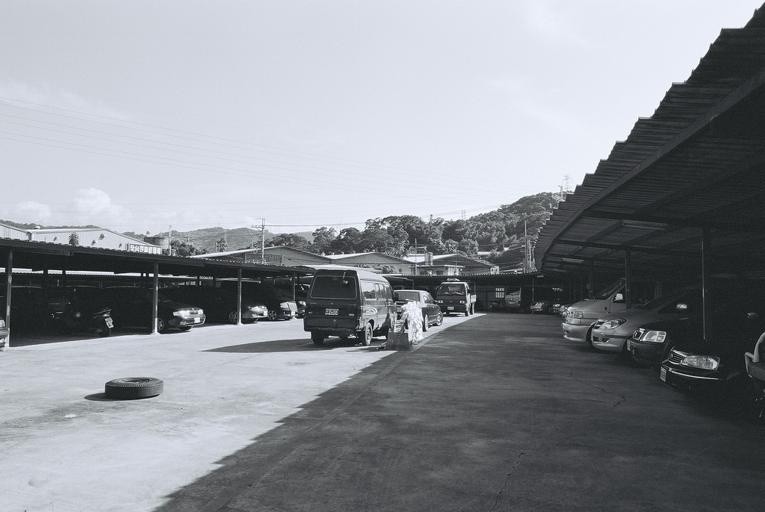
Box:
[435,282,476,316]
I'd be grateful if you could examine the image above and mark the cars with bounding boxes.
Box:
[503,272,765,398]
[394,289,443,332]
[54,285,305,337]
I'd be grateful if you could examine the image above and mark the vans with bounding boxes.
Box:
[304,267,404,346]
